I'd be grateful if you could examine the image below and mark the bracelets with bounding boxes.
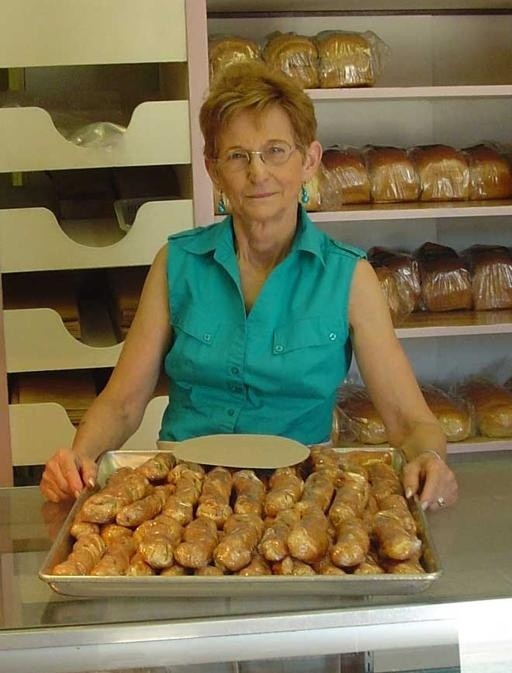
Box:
[423,449,440,459]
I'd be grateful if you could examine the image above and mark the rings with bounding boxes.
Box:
[437,496,446,507]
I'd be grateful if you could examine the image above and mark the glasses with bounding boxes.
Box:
[208,137,299,171]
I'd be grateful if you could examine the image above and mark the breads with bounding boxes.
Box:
[319,143,369,207]
[207,32,263,95]
[363,247,421,329]
[314,31,375,90]
[414,243,472,313]
[297,158,321,211]
[53,445,428,574]
[458,145,511,200]
[457,244,511,311]
[332,374,511,444]
[410,144,470,202]
[360,146,423,204]
[265,36,320,89]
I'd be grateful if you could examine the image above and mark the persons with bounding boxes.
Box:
[39,55,459,514]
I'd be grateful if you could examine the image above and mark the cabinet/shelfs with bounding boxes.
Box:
[186,1,511,454]
[0,0,194,633]
